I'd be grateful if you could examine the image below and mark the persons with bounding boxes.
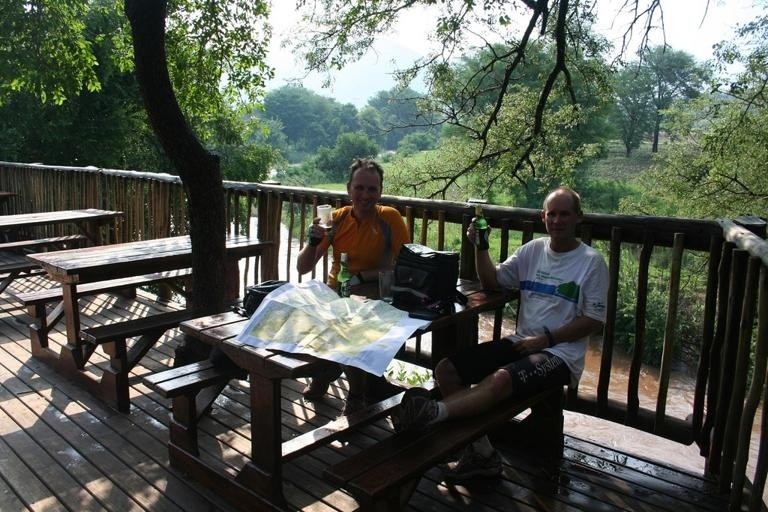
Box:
[390,185,609,483]
[292,159,412,424]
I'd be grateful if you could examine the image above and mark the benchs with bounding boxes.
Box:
[322,399,572,510]
[139,357,283,512]
[1,234,89,248]
[79,298,244,415]
[0,261,48,295]
[13,267,194,414]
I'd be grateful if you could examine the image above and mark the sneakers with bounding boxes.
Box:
[442,445,503,482]
[390,387,439,438]
[302,367,343,402]
[342,391,365,419]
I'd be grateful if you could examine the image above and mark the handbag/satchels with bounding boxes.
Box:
[242,281,290,316]
[391,244,468,321]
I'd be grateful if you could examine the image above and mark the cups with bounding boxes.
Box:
[378,270,396,304]
[316,204,332,228]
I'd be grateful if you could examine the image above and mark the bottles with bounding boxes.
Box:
[474,204,491,252]
[338,250,351,301]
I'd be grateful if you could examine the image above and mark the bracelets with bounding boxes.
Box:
[542,324,555,347]
